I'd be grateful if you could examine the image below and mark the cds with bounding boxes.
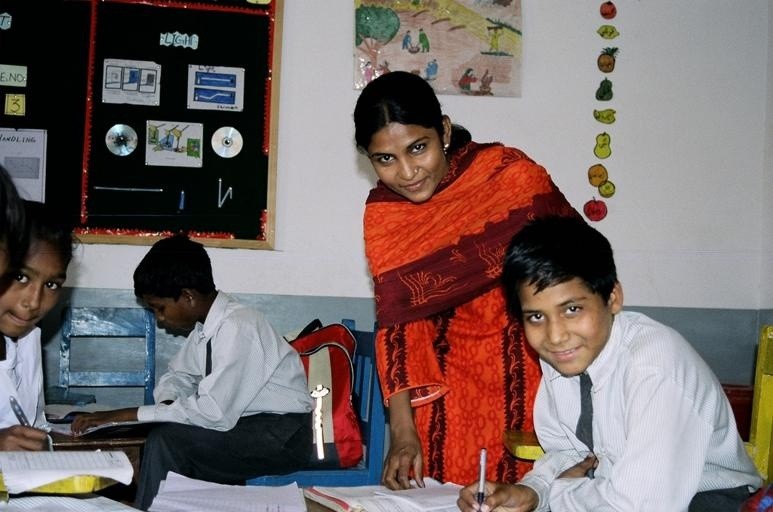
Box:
[211,126,243,159]
[105,124,138,157]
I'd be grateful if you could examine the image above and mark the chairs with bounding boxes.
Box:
[244,317,385,487]
[500,321,772,492]
[54,303,156,405]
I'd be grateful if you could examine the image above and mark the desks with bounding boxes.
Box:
[47,425,147,506]
[0,458,122,504]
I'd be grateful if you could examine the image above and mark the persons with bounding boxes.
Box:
[352,68,592,492]
[454,212,770,512]
[0,162,28,305]
[70,229,318,511]
[0,195,76,455]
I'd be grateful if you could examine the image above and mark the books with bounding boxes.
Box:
[44,415,136,436]
[374,476,474,512]
[0,449,137,497]
[301,475,432,512]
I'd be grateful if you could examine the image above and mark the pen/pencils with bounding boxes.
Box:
[9,396,31,427]
[476,448,487,512]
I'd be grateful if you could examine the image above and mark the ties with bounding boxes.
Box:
[575,371,596,480]
[203,334,215,378]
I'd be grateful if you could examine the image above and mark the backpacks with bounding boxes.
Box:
[282,318,364,469]
